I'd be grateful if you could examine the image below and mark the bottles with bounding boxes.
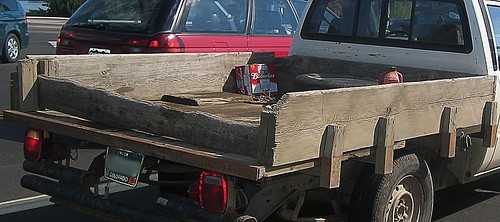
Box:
[378,65,403,85]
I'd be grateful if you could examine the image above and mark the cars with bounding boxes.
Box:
[53,0,343,59]
[0,0,30,62]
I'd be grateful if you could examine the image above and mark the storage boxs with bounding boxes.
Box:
[235,62,279,95]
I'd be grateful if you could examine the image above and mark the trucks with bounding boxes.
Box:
[2,1,499,221]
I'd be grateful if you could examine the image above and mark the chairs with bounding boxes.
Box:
[253,8,287,36]
